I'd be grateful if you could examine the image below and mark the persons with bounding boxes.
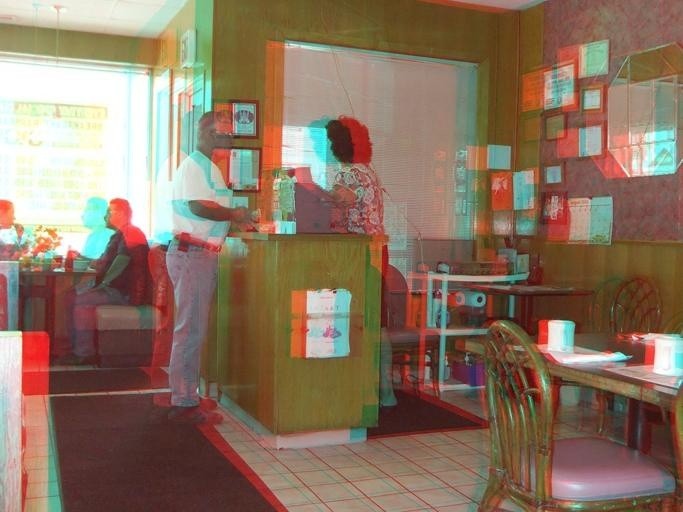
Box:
[0,198,24,332]
[61,195,155,365]
[162,107,253,425]
[292,113,399,410]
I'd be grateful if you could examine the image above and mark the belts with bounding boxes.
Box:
[175,234,222,254]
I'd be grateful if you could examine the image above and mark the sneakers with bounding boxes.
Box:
[168,399,224,425]
[60,353,101,366]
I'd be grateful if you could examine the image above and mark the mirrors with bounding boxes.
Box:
[606,42,683,177]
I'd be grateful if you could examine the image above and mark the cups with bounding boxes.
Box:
[537,319,576,353]
[653,335,683,377]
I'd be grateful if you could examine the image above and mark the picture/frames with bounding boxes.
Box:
[228,99,263,193]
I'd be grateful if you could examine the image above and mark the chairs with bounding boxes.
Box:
[94,244,172,371]
[479,273,681,510]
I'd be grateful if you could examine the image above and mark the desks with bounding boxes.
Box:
[473,283,594,336]
[459,316,682,488]
[17,261,103,358]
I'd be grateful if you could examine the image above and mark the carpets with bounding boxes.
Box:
[21,365,287,512]
[366,384,489,438]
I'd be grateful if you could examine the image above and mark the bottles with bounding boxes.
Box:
[616,332,646,364]
[64,244,74,272]
[271,167,295,222]
[31,247,54,270]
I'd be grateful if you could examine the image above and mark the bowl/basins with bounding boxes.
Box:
[73,260,89,272]
